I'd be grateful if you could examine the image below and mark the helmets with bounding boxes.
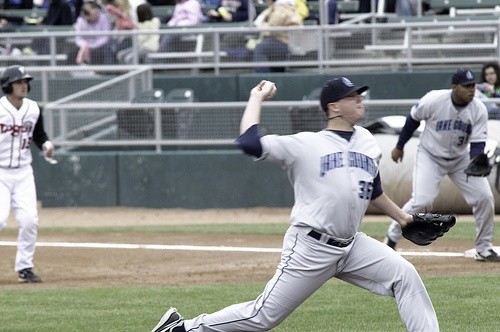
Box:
[0,64,34,87]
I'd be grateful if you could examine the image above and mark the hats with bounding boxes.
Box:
[321,77,370,107]
[452,68,481,86]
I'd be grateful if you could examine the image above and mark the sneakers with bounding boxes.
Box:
[151,307,184,332]
[474,249,500,262]
[18,268,40,282]
[385,234,397,251]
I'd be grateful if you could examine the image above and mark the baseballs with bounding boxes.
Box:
[261,81,277,97]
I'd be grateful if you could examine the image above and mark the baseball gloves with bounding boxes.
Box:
[400,213,458,247]
[464,153,493,177]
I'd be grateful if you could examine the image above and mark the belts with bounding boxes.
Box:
[442,157,457,161]
[308,230,355,248]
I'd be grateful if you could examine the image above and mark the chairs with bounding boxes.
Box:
[0,0,414,71]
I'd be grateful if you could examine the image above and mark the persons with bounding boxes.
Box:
[0,0,430,73]
[149,77,442,332]
[476,62,500,97]
[0,64,55,281]
[382,67,500,263]
